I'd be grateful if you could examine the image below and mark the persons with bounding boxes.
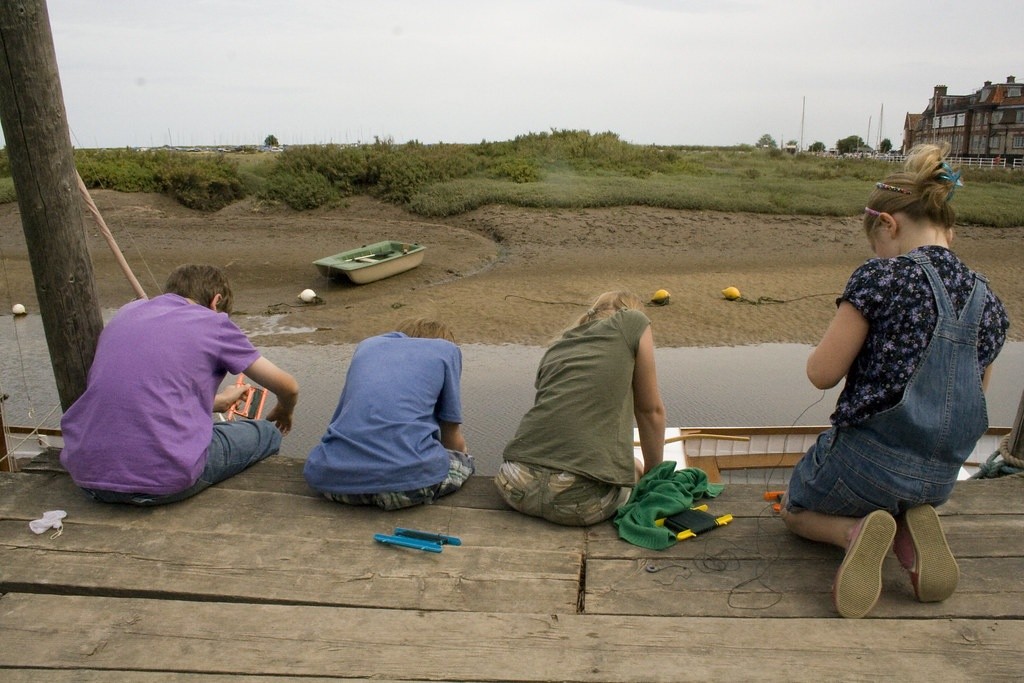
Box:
[493,291,666,527]
[303,317,476,511]
[781,139,1010,619]
[58,265,298,507]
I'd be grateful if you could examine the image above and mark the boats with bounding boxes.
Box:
[312,241,426,285]
[633,425,1012,486]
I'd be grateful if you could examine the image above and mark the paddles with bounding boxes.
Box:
[342,250,394,262]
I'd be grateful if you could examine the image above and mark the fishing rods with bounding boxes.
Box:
[71,168,151,302]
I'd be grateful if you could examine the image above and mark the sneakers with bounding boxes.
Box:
[835,510,897,618]
[893,504,959,603]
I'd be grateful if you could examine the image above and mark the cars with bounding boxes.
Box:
[261,146,283,152]
